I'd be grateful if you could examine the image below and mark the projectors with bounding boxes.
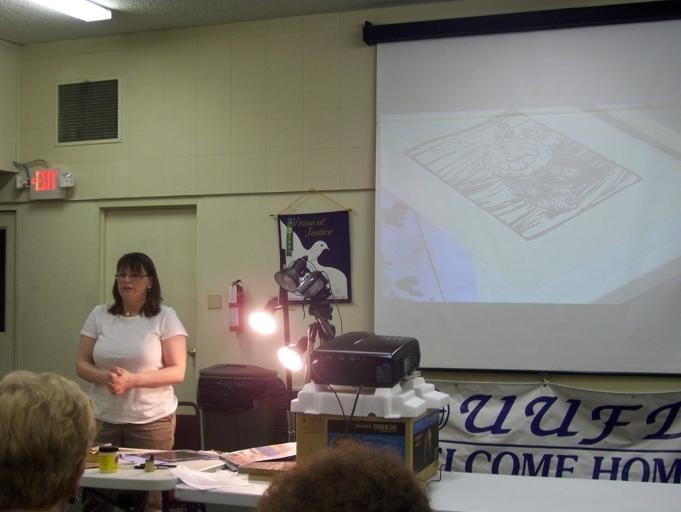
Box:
[311,329,420,389]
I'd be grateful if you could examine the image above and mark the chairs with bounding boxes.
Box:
[169,401,206,512]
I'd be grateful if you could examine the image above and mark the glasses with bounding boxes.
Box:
[115,271,150,280]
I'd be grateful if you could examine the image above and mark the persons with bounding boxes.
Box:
[71,250,190,512]
[0,369,95,511]
[249,439,436,512]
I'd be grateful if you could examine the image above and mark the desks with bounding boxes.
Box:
[70,445,681,512]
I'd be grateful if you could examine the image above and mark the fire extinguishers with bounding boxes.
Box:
[229,280,244,332]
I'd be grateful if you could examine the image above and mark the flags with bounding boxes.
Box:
[277,210,353,307]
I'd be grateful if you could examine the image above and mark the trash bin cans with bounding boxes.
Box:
[198,363,278,451]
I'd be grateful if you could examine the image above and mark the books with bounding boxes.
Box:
[237,458,298,475]
[217,440,297,473]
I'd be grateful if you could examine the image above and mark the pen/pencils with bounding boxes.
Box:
[135,464,177,469]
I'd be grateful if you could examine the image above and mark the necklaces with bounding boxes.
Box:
[120,309,138,318]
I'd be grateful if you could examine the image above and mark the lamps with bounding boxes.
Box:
[245,254,336,386]
